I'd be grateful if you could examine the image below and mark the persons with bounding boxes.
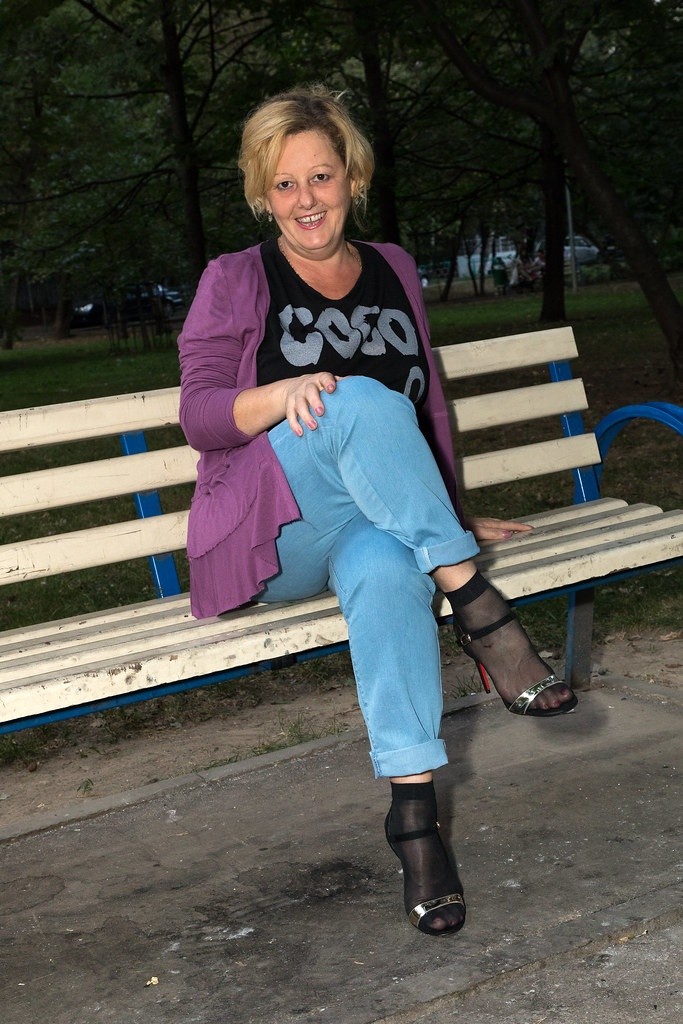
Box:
[175,86,581,937]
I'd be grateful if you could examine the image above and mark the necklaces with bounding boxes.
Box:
[278,237,362,286]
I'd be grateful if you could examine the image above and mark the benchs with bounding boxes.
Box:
[0,326,683,735]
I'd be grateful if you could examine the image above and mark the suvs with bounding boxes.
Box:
[535,234,603,268]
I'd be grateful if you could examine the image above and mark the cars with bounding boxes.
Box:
[72,279,183,326]
[417,268,431,287]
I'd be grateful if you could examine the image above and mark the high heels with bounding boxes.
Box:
[453,611,578,717]
[384,809,467,936]
